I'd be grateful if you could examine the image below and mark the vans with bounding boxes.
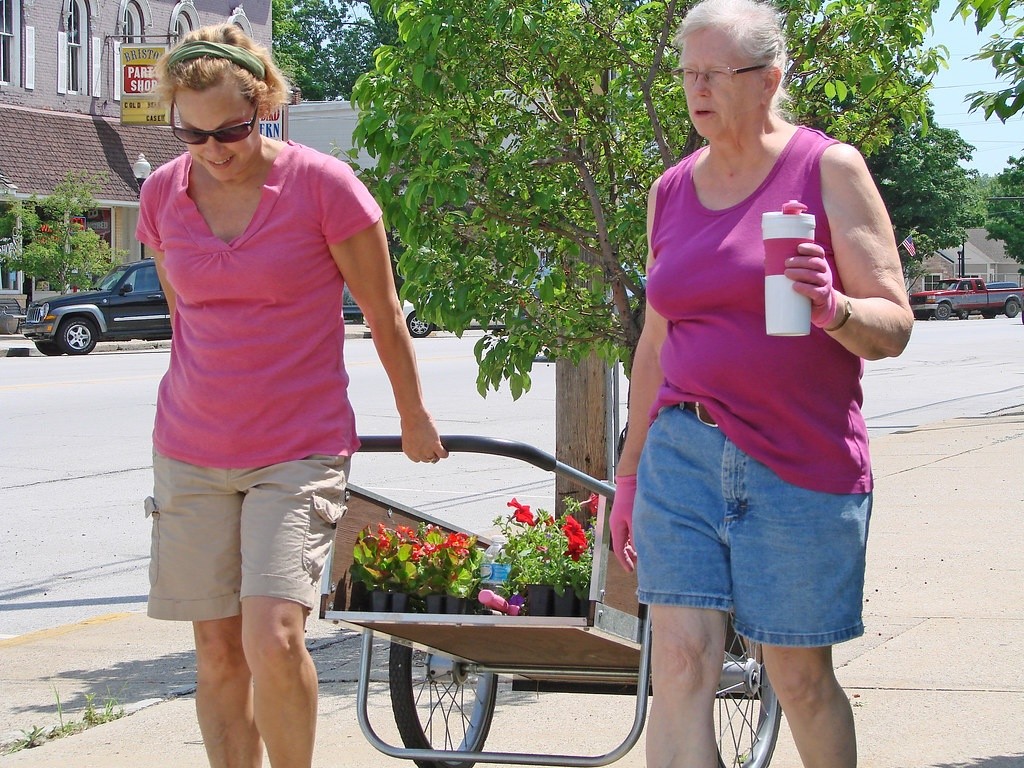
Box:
[980,282,1018,319]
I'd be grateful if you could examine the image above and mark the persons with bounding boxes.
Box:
[135,26,450,768]
[610,0,914,768]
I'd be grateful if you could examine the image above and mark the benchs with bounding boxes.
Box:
[0,297,30,336]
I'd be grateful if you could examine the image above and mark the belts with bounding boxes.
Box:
[682,400,719,427]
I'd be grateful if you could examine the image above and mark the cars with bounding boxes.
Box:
[363,290,518,338]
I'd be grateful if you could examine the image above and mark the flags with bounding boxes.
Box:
[903,236,916,256]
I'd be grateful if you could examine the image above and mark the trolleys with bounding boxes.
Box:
[317,432,784,767]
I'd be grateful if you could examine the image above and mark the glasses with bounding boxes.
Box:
[170,101,258,145]
[672,64,767,91]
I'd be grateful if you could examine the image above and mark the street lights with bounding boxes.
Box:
[132,152,151,260]
[955,244,963,278]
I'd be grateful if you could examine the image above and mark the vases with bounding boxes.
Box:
[357,579,594,619]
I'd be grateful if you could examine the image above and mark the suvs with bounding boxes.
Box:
[21,256,173,357]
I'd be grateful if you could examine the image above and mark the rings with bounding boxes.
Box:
[428,456,438,463]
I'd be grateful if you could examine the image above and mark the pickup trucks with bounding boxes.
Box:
[908,277,1024,320]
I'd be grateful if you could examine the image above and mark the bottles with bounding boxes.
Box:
[480,535,512,616]
[761,199,815,338]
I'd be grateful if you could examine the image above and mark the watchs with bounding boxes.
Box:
[823,295,853,331]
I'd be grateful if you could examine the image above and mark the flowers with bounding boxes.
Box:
[342,490,600,574]
